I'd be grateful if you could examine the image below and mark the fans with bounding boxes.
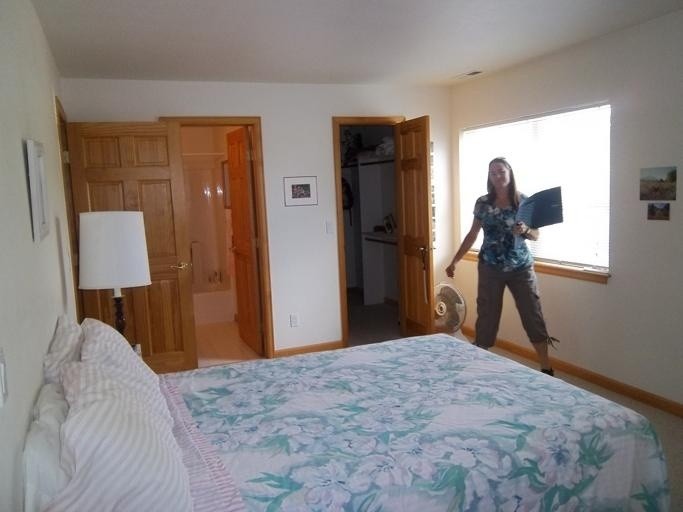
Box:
[433,281,467,333]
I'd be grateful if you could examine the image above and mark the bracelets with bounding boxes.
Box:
[522,227,532,235]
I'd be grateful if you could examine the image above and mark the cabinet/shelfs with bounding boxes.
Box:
[359,159,399,245]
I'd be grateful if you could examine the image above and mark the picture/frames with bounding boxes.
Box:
[282,176,318,207]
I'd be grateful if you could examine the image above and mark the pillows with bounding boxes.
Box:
[37,316,194,508]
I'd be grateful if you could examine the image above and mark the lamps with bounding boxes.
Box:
[73,209,153,335]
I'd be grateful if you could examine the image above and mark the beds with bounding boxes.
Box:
[22,330,675,510]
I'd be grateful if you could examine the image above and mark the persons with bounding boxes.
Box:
[444,158,560,378]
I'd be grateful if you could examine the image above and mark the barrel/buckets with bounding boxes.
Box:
[388,213,394,228]
[383,216,392,235]
[373,216,384,235]
